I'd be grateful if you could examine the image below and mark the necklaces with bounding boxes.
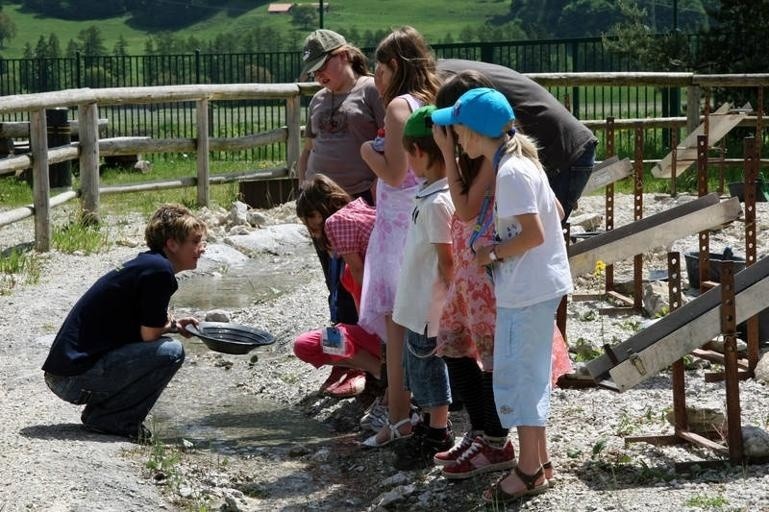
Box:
[329,72,360,119]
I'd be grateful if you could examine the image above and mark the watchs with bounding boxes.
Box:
[488,244,503,263]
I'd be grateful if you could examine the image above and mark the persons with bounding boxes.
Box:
[293,232,390,409]
[41,205,206,440]
[297,172,464,432]
[431,68,518,480]
[298,29,387,399]
[373,57,600,229]
[431,87,573,505]
[391,105,455,470]
[360,25,446,449]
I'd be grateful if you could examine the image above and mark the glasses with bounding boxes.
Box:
[311,55,337,76]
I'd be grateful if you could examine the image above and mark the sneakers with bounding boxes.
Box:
[363,396,384,413]
[442,438,515,480]
[371,410,392,431]
[433,429,483,464]
[86,423,149,439]
[359,405,388,423]
[425,419,454,451]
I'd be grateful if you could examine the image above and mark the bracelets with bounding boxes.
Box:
[449,178,462,187]
[170,319,177,333]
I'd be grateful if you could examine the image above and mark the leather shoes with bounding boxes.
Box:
[327,372,368,396]
[317,361,350,398]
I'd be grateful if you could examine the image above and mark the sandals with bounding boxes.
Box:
[481,466,538,504]
[361,418,414,447]
[540,461,556,489]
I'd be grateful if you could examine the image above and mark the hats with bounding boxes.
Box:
[403,105,437,138]
[431,89,516,137]
[301,28,347,75]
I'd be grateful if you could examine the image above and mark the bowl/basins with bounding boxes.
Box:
[184,321,277,355]
